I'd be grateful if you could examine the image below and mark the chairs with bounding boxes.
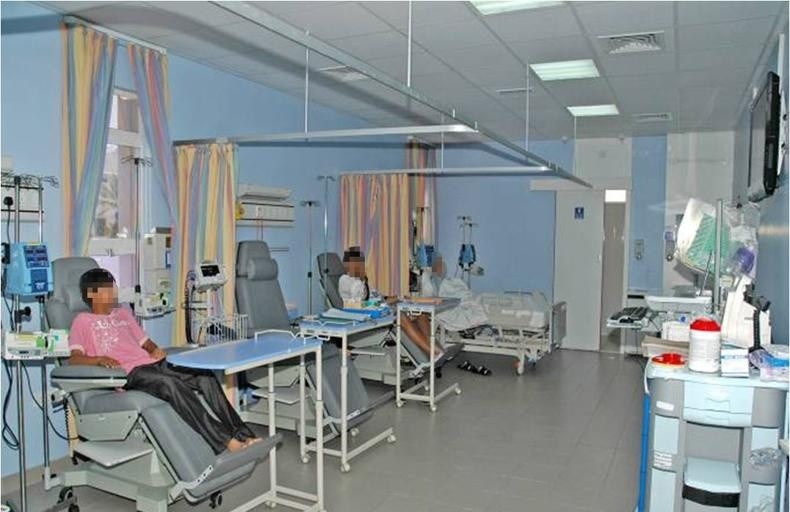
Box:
[234,240,464,440]
[39,256,282,512]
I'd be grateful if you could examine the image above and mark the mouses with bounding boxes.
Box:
[620,318,634,323]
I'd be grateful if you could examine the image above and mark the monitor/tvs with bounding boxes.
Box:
[746,71,781,203]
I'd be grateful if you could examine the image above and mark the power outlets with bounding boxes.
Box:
[1,177,43,221]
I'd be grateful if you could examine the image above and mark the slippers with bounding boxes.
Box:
[473,364,492,376]
[457,360,474,371]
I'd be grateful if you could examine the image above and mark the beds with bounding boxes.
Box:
[410,267,568,376]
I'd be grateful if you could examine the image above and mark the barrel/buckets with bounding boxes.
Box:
[690,316,721,372]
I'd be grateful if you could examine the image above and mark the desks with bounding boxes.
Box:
[166,297,462,512]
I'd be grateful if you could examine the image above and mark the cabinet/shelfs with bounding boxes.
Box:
[641,294,790,512]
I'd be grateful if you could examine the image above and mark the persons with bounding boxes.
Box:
[67,267,262,454]
[425,254,503,341]
[337,246,448,357]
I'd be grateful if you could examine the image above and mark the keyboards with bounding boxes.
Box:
[629,305,647,320]
